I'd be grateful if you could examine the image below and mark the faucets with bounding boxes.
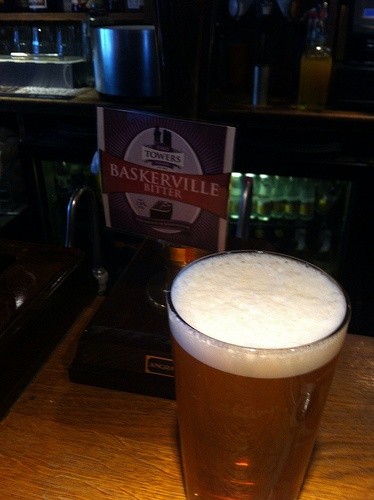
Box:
[64,186,105,274]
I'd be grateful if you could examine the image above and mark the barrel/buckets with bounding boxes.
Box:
[88,22,159,107]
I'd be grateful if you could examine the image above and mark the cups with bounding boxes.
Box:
[164,250,351,500]
[253,63,272,107]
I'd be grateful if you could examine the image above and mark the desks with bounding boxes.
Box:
[0,295,373,500]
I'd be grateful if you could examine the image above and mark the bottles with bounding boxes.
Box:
[26,0,48,13]
[297,1,334,114]
[228,172,339,222]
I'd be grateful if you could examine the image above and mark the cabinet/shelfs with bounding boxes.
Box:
[0,0,166,238]
[209,97,374,335]
